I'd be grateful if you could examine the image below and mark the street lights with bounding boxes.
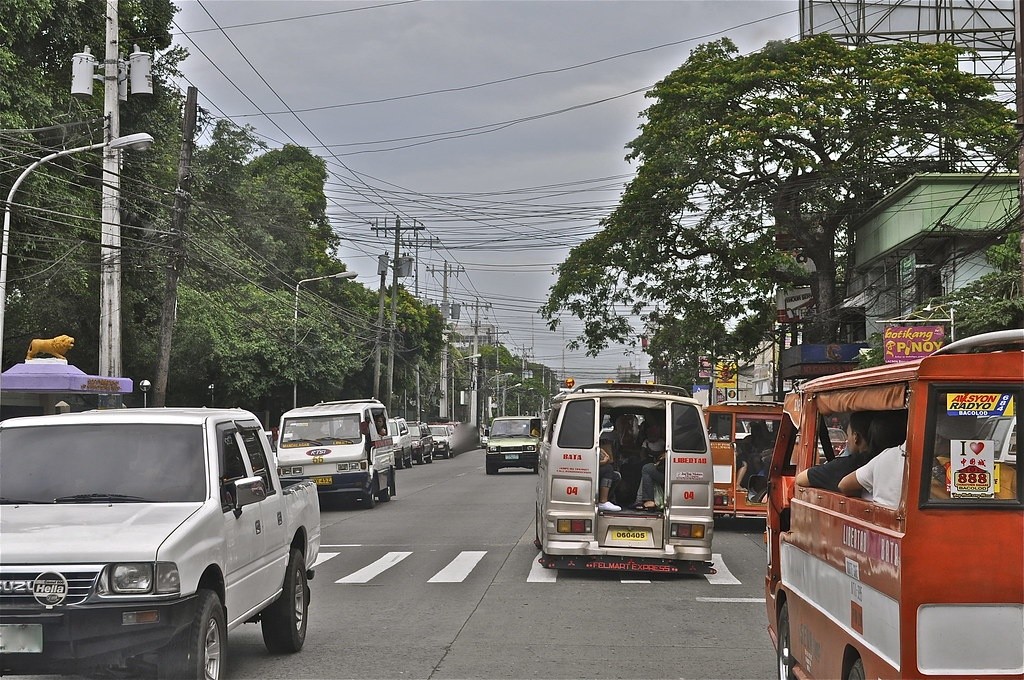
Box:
[452,353,482,422]
[503,383,522,416]
[292,271,359,409]
[482,372,514,424]
[518,387,533,416]
[0,131,155,374]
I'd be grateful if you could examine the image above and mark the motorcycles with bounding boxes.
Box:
[704,400,784,519]
[746,330,1024,680]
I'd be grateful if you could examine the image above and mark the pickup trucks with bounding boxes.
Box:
[0,407,322,680]
[427,424,453,460]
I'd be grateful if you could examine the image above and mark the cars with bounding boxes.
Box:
[429,422,461,435]
[816,427,848,466]
[481,428,489,449]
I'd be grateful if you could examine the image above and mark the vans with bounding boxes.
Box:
[528,382,717,575]
[272,397,395,510]
[263,430,279,453]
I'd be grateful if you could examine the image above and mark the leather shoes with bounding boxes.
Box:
[635,503,656,512]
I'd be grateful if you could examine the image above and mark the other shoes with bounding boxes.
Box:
[736,486,747,492]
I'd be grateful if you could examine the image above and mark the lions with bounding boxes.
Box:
[26,335,74,358]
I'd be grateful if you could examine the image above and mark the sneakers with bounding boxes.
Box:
[599,502,621,512]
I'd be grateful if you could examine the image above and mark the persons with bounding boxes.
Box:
[599,411,665,513]
[294,415,387,442]
[531,423,541,437]
[795,411,977,511]
[736,421,772,492]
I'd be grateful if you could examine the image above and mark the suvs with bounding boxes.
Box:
[386,416,414,470]
[485,416,543,474]
[406,420,435,466]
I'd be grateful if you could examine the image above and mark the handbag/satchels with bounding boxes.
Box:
[635,464,664,510]
